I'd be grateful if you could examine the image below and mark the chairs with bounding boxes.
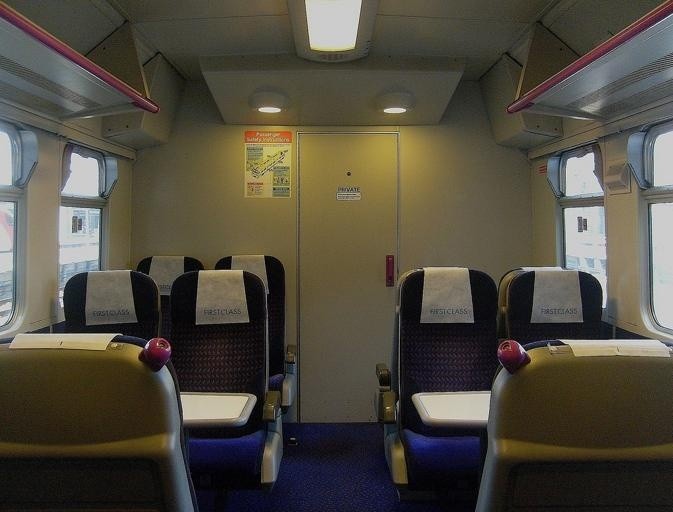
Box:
[1,332,197,509]
[166,267,285,508]
[371,268,672,511]
[135,255,205,324]
[64,269,161,344]
[213,254,296,453]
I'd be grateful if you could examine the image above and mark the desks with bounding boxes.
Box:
[180,390,257,463]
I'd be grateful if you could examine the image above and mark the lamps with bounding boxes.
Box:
[283,0,378,62]
